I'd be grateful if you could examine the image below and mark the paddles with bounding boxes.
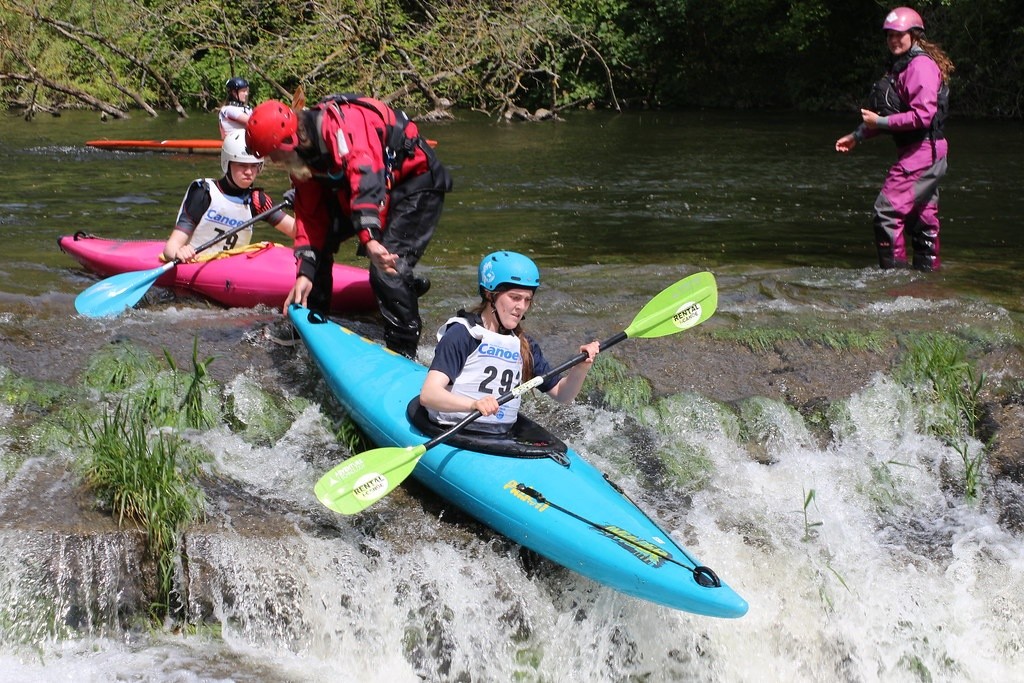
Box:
[291,85,307,115]
[72,199,290,321]
[311,268,722,516]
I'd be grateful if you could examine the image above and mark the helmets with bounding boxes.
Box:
[882,7,925,33]
[478,250,540,295]
[245,100,299,160]
[221,128,264,176]
[225,77,249,94]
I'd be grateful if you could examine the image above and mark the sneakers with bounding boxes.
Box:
[264,318,303,352]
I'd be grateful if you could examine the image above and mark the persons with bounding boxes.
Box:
[245,97,453,359]
[835,7,955,270]
[420,251,600,434]
[163,128,296,265]
[219,77,253,140]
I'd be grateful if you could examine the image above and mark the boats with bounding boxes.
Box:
[57,229,431,316]
[85,137,225,154]
[287,301,749,618]
